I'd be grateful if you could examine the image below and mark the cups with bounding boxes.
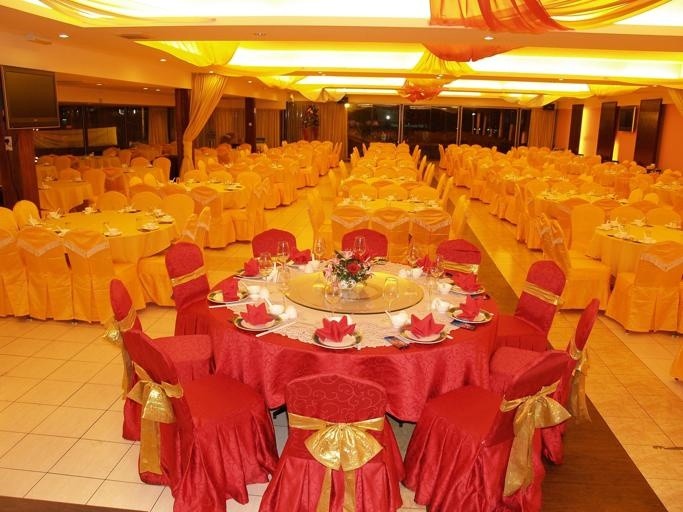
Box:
[426,195,433,208]
[331,316,341,322]
[269,304,285,317]
[313,237,326,267]
[163,215,170,219]
[339,314,353,326]
[285,305,298,318]
[146,222,152,227]
[411,268,424,279]
[276,241,290,270]
[408,201,415,213]
[382,277,399,313]
[136,217,144,231]
[257,252,275,281]
[618,224,630,238]
[397,311,409,320]
[306,264,313,273]
[308,261,320,270]
[385,198,392,207]
[438,283,451,295]
[390,315,406,329]
[275,269,293,313]
[108,228,118,234]
[643,227,656,243]
[260,287,270,299]
[353,237,366,260]
[671,215,682,232]
[248,286,260,299]
[432,298,441,310]
[429,254,444,287]
[360,197,369,208]
[425,276,441,310]
[85,208,91,212]
[436,302,450,314]
[324,280,342,316]
[406,243,422,272]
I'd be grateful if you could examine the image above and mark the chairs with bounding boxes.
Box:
[607,241,683,338]
[412,207,453,253]
[253,162,281,208]
[357,159,376,167]
[438,144,447,169]
[84,170,106,195]
[331,205,371,259]
[171,207,216,255]
[313,144,334,175]
[373,208,412,249]
[351,146,360,169]
[20,225,70,321]
[348,185,375,199]
[194,148,218,169]
[333,144,338,153]
[0,226,29,317]
[308,196,334,259]
[525,198,554,251]
[236,173,260,207]
[375,166,397,178]
[63,228,145,324]
[489,295,602,392]
[98,190,127,212]
[629,188,644,205]
[339,160,349,180]
[376,160,396,169]
[452,194,472,238]
[209,171,232,182]
[102,155,122,167]
[132,191,161,211]
[579,182,607,196]
[351,166,372,178]
[165,242,213,336]
[524,180,550,195]
[183,170,208,183]
[277,158,300,204]
[432,238,486,283]
[140,225,210,309]
[249,227,298,261]
[396,159,415,169]
[471,161,486,198]
[412,145,419,161]
[162,193,196,214]
[488,173,513,219]
[131,157,152,168]
[410,187,440,200]
[546,216,604,317]
[296,149,318,189]
[516,184,530,244]
[647,207,681,227]
[362,143,368,157]
[329,142,343,168]
[339,227,389,263]
[153,158,171,182]
[398,168,418,178]
[612,206,646,227]
[328,169,343,199]
[629,174,654,192]
[416,155,427,181]
[226,180,263,244]
[491,259,568,350]
[436,173,447,199]
[570,202,605,263]
[378,184,409,202]
[106,275,216,443]
[453,155,469,186]
[643,192,660,204]
[414,149,420,167]
[59,167,82,181]
[119,326,277,510]
[550,182,577,194]
[407,348,579,511]
[656,175,677,185]
[440,177,456,208]
[16,197,41,228]
[425,166,436,186]
[447,149,454,175]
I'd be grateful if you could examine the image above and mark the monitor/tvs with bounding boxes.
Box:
[1,65,61,130]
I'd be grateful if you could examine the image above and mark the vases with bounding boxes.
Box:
[337,277,356,289]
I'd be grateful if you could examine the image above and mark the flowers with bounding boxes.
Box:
[259,370,404,511]
[319,248,375,288]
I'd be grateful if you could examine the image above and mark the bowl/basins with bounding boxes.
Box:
[49,212,58,218]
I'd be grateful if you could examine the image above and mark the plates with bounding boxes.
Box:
[446,306,492,324]
[448,283,486,295]
[140,226,159,231]
[103,232,122,236]
[311,329,364,350]
[233,313,285,332]
[286,259,308,268]
[159,219,173,223]
[400,327,450,346]
[207,289,250,304]
[233,268,264,280]
